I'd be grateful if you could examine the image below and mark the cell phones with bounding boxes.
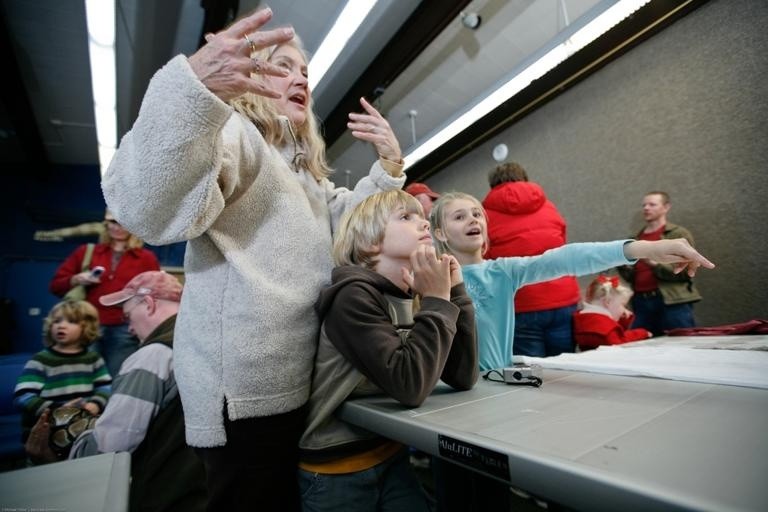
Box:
[89,266,106,279]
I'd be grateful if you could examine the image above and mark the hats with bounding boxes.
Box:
[98,270,183,307]
[405,182,441,201]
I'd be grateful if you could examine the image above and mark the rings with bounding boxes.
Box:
[244,33,255,59]
[252,57,260,74]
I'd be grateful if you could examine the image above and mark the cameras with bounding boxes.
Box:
[502,360,542,386]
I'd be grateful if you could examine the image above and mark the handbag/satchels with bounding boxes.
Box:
[63,285,86,300]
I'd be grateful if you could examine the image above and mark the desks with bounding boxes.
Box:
[1,446,133,511]
[336,327,766,510]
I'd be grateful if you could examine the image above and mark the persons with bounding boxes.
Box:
[483,163,580,358]
[13,298,115,466]
[293,187,480,512]
[100,6,407,511]
[67,269,183,461]
[406,181,440,222]
[430,191,716,386]
[47,205,161,382]
[572,271,654,351]
[617,191,704,334]
